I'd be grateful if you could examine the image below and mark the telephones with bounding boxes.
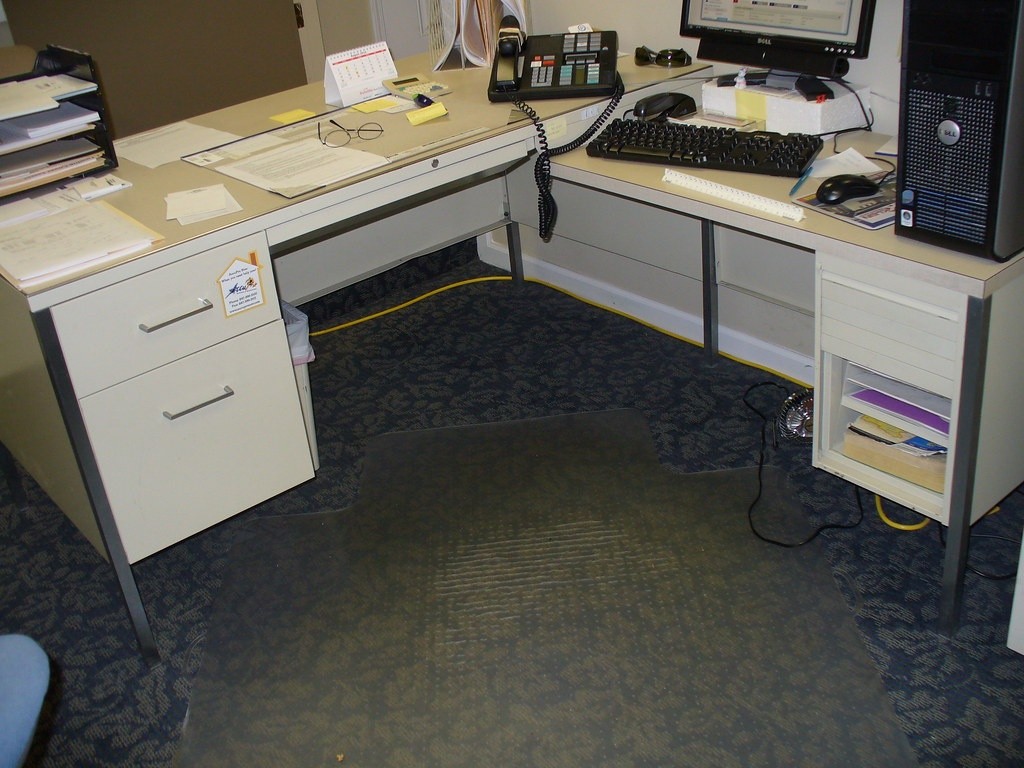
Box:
[486,14,619,102]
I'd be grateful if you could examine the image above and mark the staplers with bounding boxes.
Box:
[633,92,696,123]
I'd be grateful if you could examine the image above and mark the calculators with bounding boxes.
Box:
[381,72,453,101]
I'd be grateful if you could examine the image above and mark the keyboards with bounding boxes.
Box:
[585,118,823,177]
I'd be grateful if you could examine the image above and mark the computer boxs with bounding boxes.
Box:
[894,0,1024,262]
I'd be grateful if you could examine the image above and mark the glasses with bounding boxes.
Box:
[635,46,692,68]
[415,93,448,117]
[318,120,384,148]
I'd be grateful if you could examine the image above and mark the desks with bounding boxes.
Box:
[0,24,711,670]
[475,49,1022,632]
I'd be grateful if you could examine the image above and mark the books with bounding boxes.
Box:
[0,74,104,191]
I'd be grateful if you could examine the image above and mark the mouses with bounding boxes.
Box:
[816,174,880,205]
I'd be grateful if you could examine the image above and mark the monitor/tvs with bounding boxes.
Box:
[679,0,876,101]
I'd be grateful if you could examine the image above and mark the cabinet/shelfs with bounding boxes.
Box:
[811,199,1024,631]
[0,133,320,669]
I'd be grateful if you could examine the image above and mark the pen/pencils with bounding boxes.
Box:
[788,165,813,196]
